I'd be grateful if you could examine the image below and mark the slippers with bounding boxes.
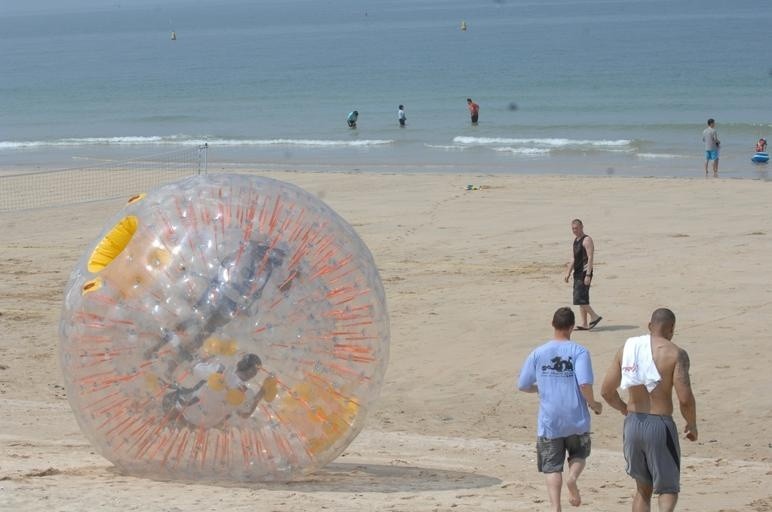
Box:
[574,325,588,330]
[587,316,602,329]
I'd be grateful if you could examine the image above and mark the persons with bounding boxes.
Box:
[598,307,700,512]
[755,137,766,152]
[565,217,602,332]
[466,98,479,124]
[160,350,273,430]
[517,306,602,512]
[346,110,359,128]
[701,119,721,175]
[397,104,407,127]
